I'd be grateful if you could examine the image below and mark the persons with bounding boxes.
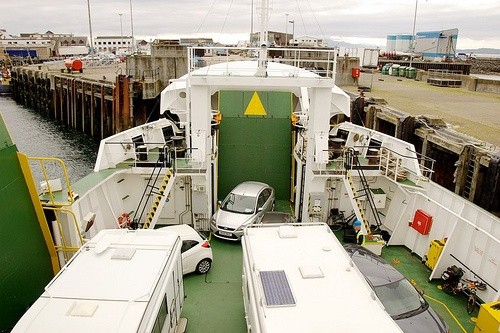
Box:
[360,90,366,97]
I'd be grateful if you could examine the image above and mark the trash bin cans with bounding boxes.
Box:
[382,64,416,79]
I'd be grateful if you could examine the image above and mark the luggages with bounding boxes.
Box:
[343,228,357,243]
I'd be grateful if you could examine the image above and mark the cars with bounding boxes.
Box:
[155,224,213,276]
[339,242,450,333]
[210,181,276,241]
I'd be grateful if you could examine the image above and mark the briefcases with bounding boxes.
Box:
[330,208,339,215]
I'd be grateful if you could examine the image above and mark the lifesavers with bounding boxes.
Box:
[120,213,130,229]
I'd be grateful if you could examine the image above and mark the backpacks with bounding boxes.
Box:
[440,265,465,288]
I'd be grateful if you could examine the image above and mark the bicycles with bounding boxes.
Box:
[326,208,357,232]
[442,265,487,315]
